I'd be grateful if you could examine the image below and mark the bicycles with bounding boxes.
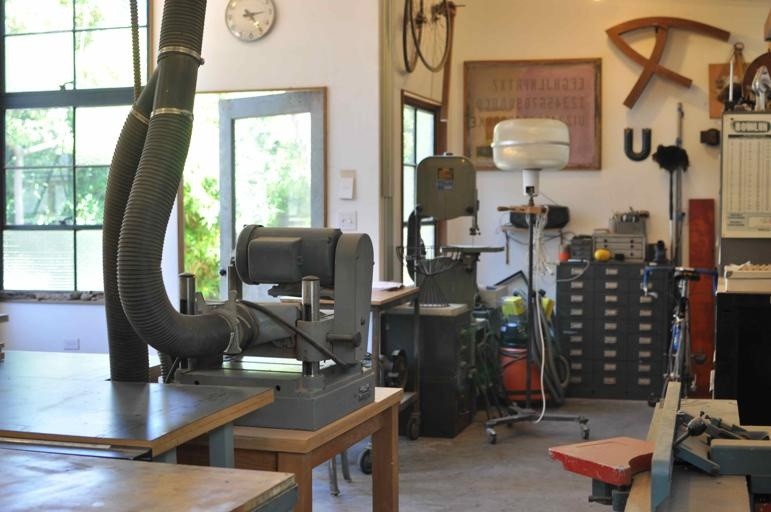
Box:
[644,266,716,399]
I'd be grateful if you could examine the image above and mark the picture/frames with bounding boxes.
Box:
[463,58,601,170]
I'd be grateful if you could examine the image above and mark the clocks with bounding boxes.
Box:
[226,0,275,42]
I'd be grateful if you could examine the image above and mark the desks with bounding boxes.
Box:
[179,386,405,511]
[0,350,275,458]
[0,444,298,511]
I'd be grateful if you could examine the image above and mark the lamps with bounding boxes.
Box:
[485,119,590,444]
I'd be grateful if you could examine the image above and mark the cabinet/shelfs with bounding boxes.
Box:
[382,302,472,438]
[555,262,673,402]
[592,230,646,263]
